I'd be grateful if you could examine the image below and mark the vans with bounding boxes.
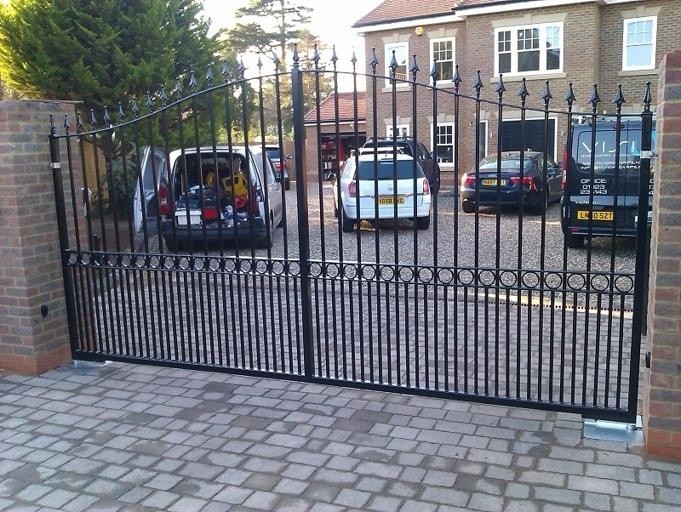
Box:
[133,144,289,252]
[559,115,656,249]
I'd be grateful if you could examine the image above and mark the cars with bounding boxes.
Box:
[257,145,294,190]
[460,150,561,214]
[333,135,440,232]
